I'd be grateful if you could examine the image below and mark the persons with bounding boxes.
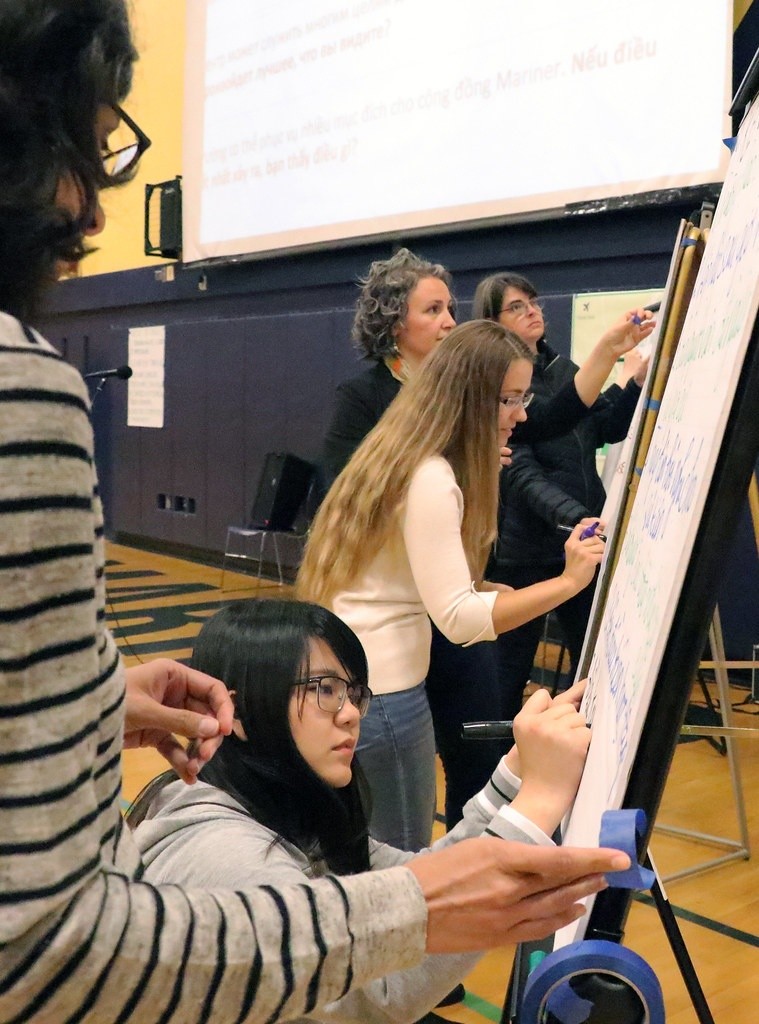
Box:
[123,599,592,1024]
[0,0,631,1024]
[323,247,658,686]
[293,318,605,853]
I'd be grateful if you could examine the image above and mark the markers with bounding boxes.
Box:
[461,720,592,744]
[562,522,600,559]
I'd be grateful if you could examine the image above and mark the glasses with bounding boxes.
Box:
[291,675,372,720]
[102,95,151,188]
[499,393,534,409]
[499,296,545,313]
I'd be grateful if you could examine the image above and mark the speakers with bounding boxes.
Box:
[161,184,183,259]
[248,450,315,534]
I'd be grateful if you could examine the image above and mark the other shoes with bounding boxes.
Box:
[435,982,465,1008]
[413,1011,463,1024]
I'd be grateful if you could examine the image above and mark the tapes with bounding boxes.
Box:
[520,940,665,1024]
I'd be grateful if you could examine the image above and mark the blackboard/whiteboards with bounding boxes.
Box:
[546,47,759,955]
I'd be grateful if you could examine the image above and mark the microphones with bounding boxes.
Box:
[84,365,133,381]
